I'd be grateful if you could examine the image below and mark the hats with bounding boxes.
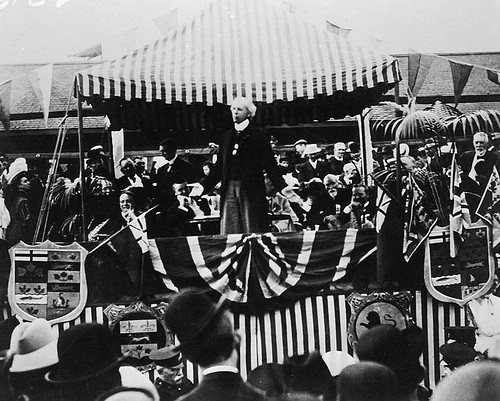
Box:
[294,139,307,146]
[356,325,427,384]
[393,143,409,158]
[302,143,322,155]
[149,346,184,368]
[44,322,129,384]
[164,289,228,352]
[4,318,59,373]
[283,352,332,386]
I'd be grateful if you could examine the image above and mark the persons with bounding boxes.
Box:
[0,132,500,401]
[186,97,299,236]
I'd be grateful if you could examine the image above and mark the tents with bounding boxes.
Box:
[71,0,402,245]
